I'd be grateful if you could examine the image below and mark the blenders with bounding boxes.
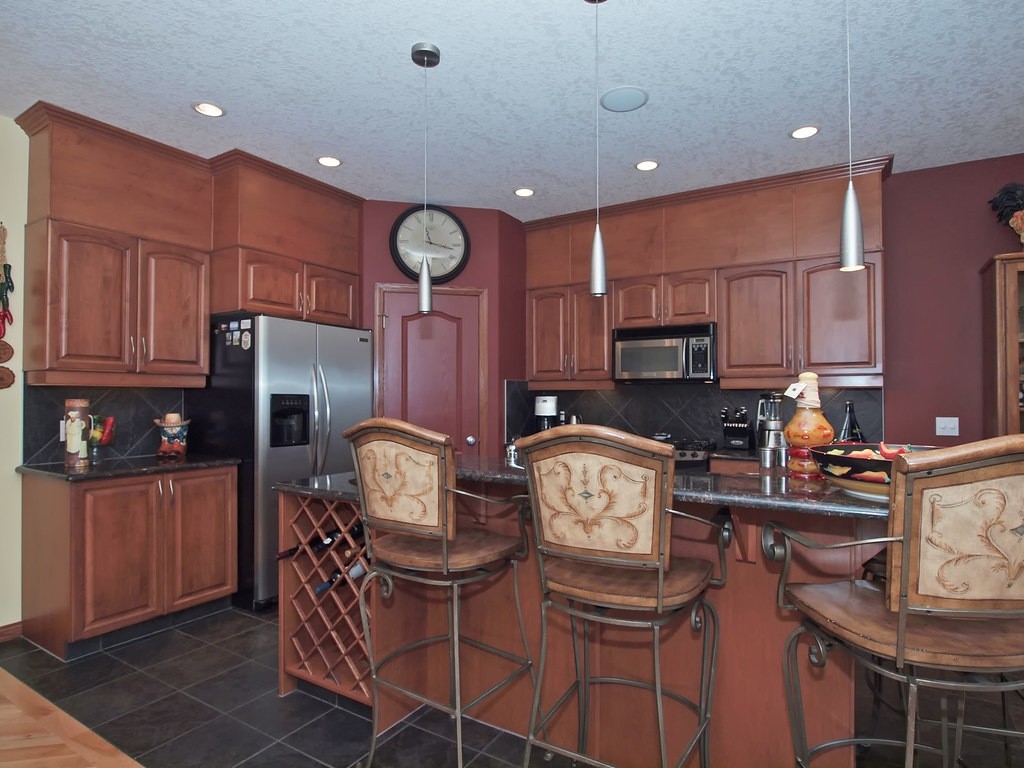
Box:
[755,392,789,451]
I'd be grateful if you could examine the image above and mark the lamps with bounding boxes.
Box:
[586,0,607,296]
[410,44,440,314]
[840,0,866,271]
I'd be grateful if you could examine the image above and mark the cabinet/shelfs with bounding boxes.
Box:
[979,252,1024,439]
[17,101,892,706]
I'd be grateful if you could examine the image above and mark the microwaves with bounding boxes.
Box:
[611,321,720,385]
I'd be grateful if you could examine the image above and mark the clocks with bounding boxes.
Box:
[389,204,471,285]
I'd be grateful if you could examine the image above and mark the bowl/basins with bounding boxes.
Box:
[807,442,948,494]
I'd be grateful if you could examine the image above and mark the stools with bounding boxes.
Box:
[760,435,1024,768]
[510,424,716,768]
[342,419,553,768]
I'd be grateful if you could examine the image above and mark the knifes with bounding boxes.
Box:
[720,406,748,438]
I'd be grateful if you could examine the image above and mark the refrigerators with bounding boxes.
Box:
[186,312,376,614]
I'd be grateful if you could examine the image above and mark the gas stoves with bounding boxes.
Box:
[644,432,718,466]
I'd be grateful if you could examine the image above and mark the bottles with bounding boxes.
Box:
[540,409,583,431]
[839,400,867,444]
